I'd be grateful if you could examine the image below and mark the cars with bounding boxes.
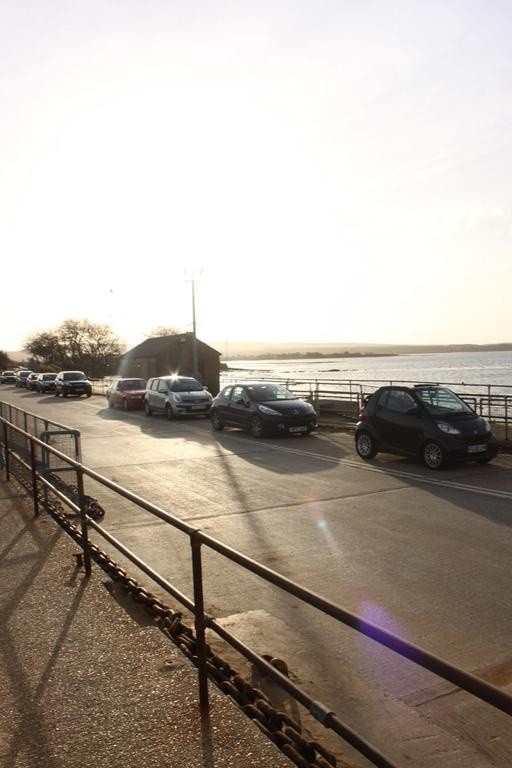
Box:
[355,383,498,471]
[143,375,213,420]
[26,373,41,390]
[16,371,33,388]
[55,370,92,397]
[107,378,147,410]
[2,371,17,383]
[36,373,58,392]
[209,382,318,437]
